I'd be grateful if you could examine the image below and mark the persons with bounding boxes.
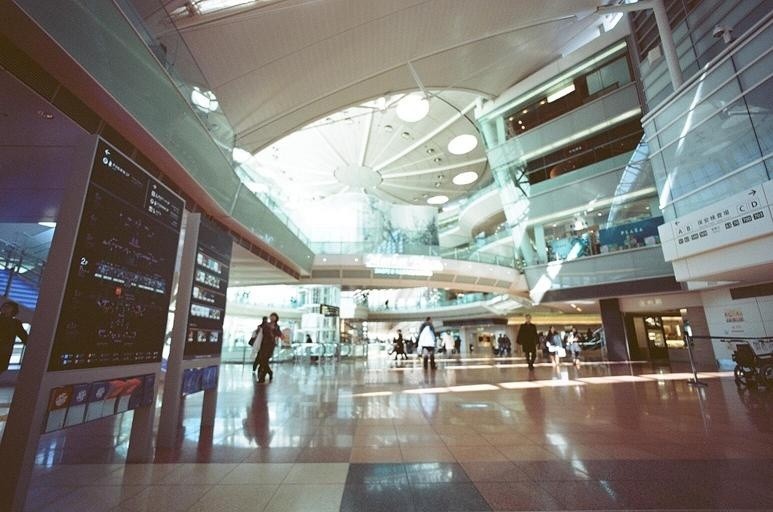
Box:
[253,313,284,383]
[517,314,539,370]
[305,334,311,343]
[0,301,29,374]
[416,316,438,370]
[387,326,600,365]
[248,316,268,375]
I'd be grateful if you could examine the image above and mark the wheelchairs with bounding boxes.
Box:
[727,342,772,390]
